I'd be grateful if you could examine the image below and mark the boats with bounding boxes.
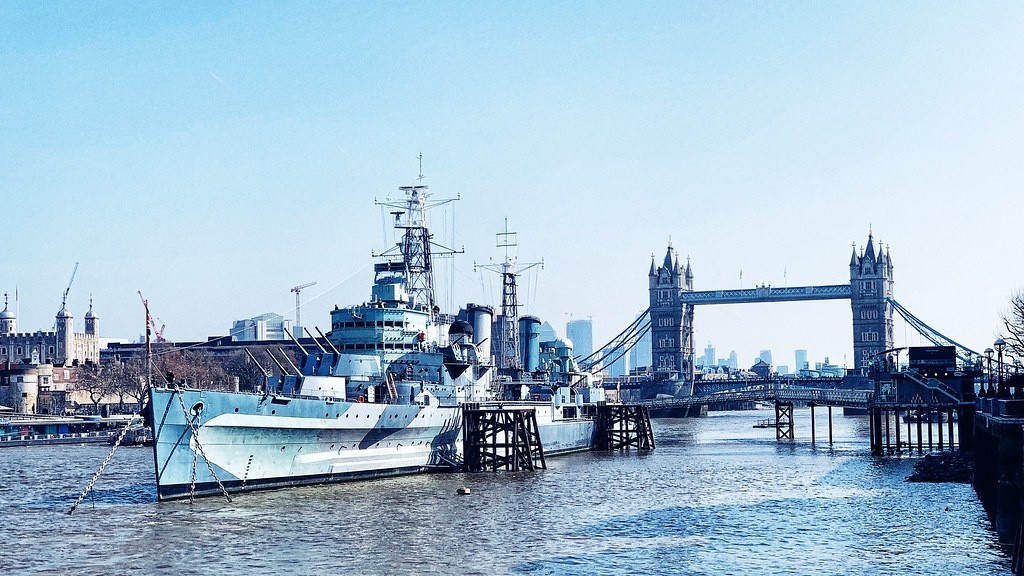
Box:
[137,152,605,503]
[107,426,153,447]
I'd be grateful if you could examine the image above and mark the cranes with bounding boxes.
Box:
[138,291,166,342]
[50,262,79,333]
[290,281,317,339]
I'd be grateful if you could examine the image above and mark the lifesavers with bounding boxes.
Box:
[357,395,365,403]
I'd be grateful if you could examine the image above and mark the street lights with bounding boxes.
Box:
[984,348,994,399]
[976,353,987,397]
[994,340,1006,399]
